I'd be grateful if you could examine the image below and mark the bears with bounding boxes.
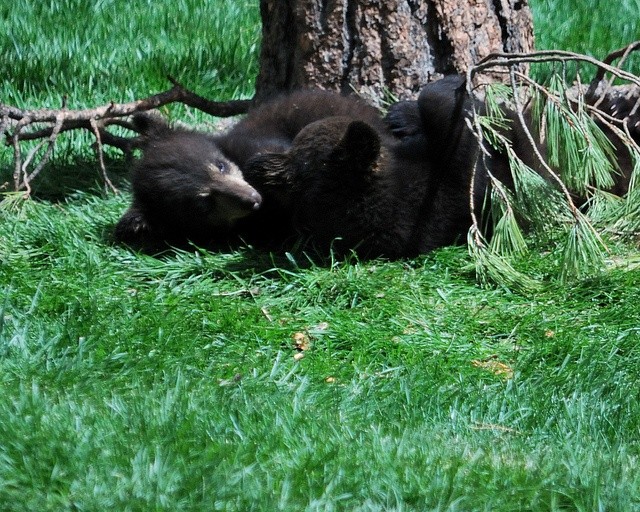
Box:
[243,74,595,269]
[108,87,387,262]
